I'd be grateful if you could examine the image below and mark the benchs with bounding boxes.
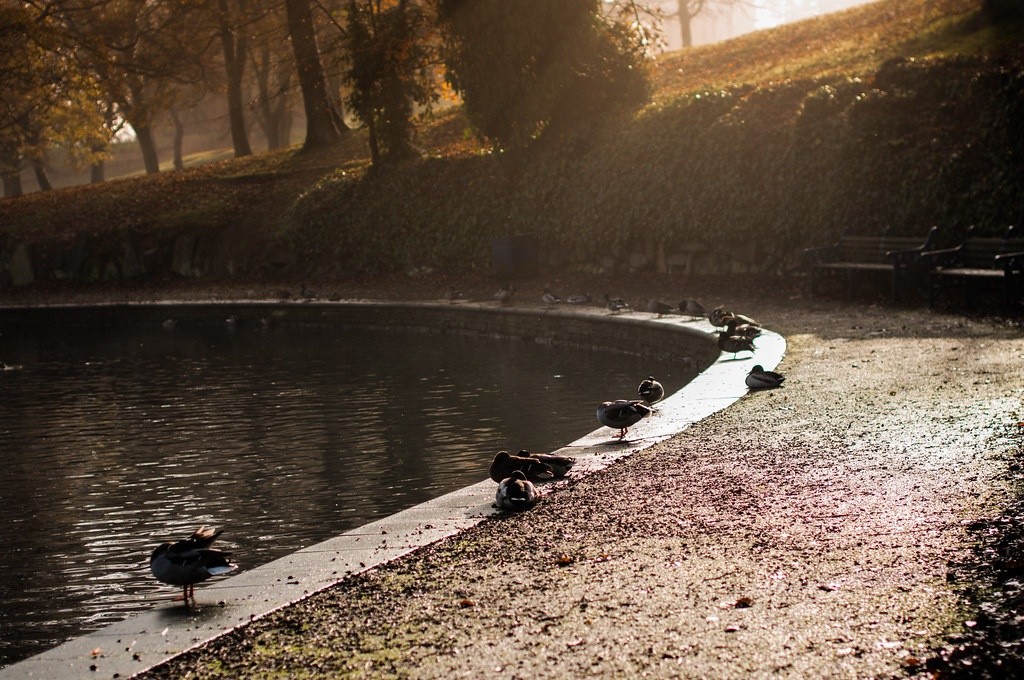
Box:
[802,226,943,303]
[918,224,1024,315]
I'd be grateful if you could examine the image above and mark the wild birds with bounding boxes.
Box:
[603,292,632,315]
[274,282,342,304]
[745,365,788,388]
[486,449,578,513]
[566,292,592,309]
[596,375,665,445]
[150,525,238,608]
[488,282,518,307]
[709,305,762,360]
[539,288,562,309]
[647,296,709,322]
[444,283,465,303]
